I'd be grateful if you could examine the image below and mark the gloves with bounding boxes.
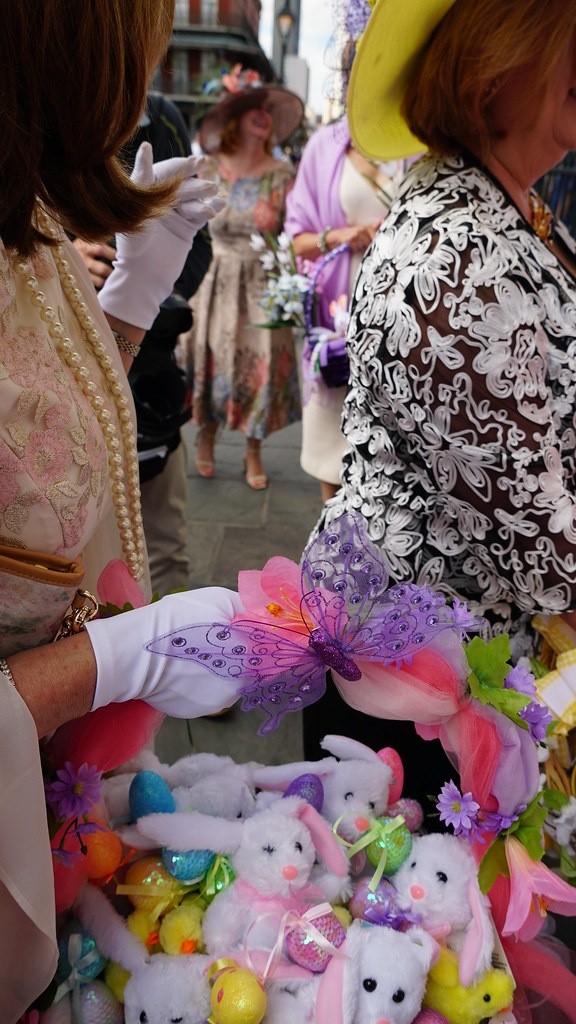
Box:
[83,586,255,718]
[95,141,228,331]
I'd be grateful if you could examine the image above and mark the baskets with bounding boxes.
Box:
[305,243,351,389]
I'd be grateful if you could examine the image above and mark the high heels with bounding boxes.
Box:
[194,429,215,477]
[243,447,268,489]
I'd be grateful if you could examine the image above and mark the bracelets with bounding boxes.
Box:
[0,659,15,690]
[317,226,332,254]
[111,329,140,357]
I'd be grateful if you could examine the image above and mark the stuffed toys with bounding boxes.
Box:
[60,735,515,1024]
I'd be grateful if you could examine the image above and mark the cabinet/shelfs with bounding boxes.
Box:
[151,0,283,120]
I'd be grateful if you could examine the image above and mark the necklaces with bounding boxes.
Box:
[9,198,146,579]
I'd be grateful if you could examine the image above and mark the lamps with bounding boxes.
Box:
[278,0,294,79]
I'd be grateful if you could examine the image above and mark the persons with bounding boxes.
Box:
[303,0,575,833]
[0,1,258,1023]
[65,35,423,600]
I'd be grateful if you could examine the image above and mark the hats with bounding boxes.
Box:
[346,0,455,161]
[200,88,304,154]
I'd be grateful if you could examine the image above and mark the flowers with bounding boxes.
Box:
[436,594,553,844]
[198,63,262,96]
